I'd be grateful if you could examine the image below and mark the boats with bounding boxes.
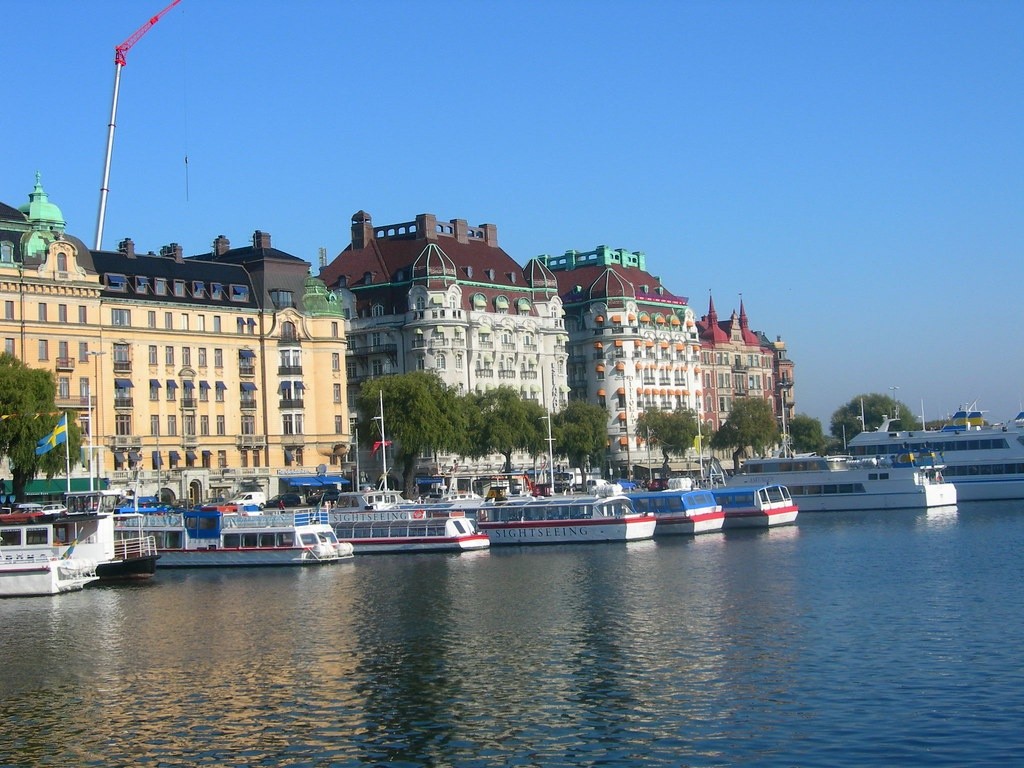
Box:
[676,395,799,530]
[591,426,725,537]
[474,401,657,545]
[82,389,354,568]
[694,397,958,513]
[0,389,159,598]
[840,392,1024,500]
[303,388,492,554]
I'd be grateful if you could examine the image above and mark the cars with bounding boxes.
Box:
[171,500,192,510]
[267,494,302,508]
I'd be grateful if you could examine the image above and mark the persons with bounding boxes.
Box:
[278,499,285,513]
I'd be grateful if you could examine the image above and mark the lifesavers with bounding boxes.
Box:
[936,475,944,483]
[413,509,424,519]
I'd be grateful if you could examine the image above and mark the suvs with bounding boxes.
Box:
[307,488,342,505]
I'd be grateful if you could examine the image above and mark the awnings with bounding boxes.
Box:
[241,382,258,391]
[280,476,351,488]
[237,317,257,325]
[114,452,139,462]
[195,282,222,293]
[151,379,179,389]
[184,381,227,390]
[285,450,294,461]
[594,316,704,446]
[412,296,572,392]
[151,451,213,466]
[115,379,134,388]
[240,350,256,358]
[280,381,305,389]
[234,287,248,293]
[108,276,150,286]
[0,478,107,495]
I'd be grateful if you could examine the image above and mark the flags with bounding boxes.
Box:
[35,416,67,456]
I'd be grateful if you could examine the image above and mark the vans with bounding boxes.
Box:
[226,491,267,510]
[118,496,158,508]
